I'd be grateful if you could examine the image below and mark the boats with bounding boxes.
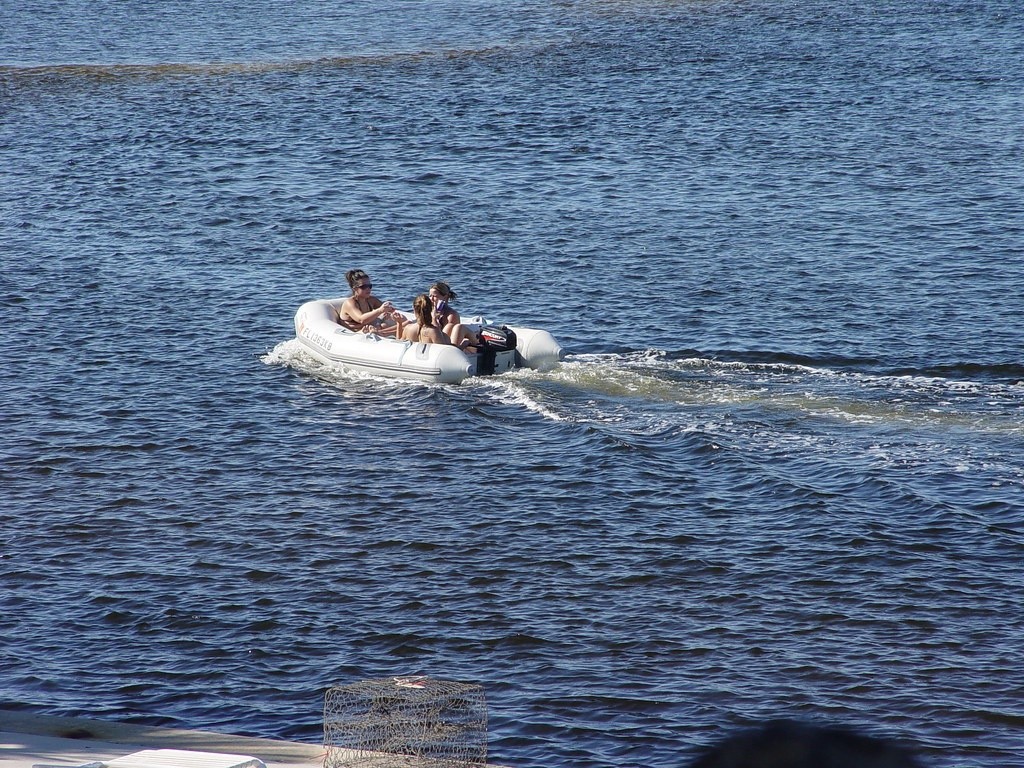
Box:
[292,299,567,384]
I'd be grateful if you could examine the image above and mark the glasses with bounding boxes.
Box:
[356,284,373,289]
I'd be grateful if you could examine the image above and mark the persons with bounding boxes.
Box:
[339,269,479,354]
